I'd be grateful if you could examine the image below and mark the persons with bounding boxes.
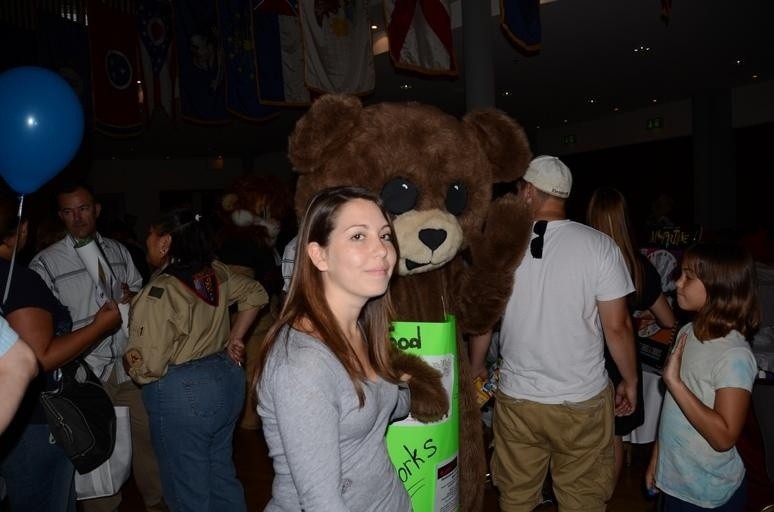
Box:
[2,152,774,510]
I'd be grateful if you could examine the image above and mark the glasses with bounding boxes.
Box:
[530,219,548,260]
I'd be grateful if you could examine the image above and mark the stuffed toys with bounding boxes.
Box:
[286,92,536,510]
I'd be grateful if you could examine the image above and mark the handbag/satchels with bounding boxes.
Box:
[74,407,132,500]
[37,359,116,474]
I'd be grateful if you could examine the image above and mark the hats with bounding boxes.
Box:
[524,155,572,199]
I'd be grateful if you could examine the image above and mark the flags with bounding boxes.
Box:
[81,2,543,140]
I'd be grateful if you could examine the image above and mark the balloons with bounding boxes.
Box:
[1,65,85,198]
